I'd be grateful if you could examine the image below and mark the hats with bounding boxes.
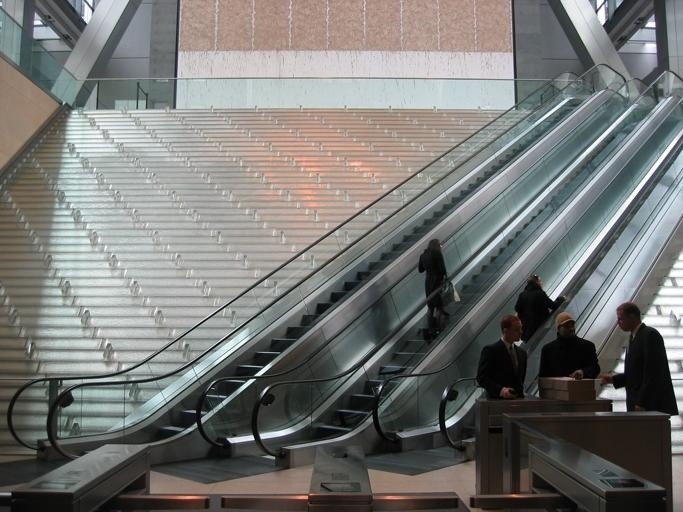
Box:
[555,312,575,329]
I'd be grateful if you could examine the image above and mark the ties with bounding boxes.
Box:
[509,345,519,365]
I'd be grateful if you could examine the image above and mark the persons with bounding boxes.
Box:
[475,314,529,400]
[538,311,601,381]
[515,274,568,344]
[596,301,680,416]
[417,238,447,329]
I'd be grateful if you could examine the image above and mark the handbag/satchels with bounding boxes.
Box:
[442,287,460,307]
